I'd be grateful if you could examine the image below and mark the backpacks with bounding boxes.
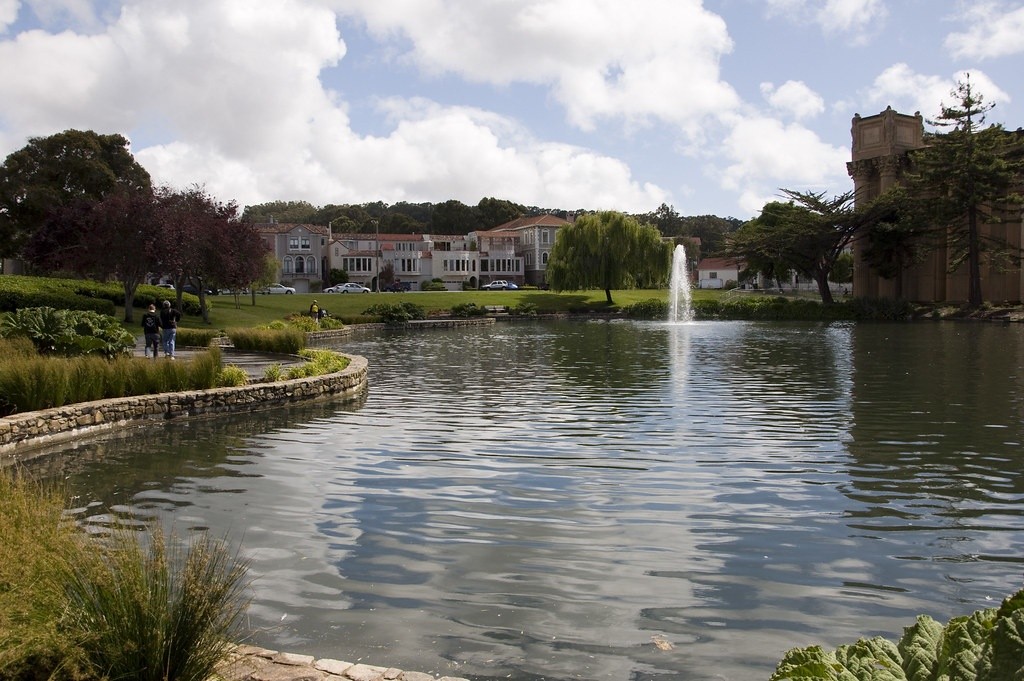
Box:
[312,304,318,312]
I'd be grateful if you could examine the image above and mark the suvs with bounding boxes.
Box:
[480,280,509,291]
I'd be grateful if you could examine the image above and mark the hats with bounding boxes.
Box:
[149,305,156,313]
[164,301,170,307]
[314,300,317,302]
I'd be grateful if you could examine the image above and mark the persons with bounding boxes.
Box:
[141,304,162,359]
[753,277,758,289]
[160,300,181,360]
[310,300,319,318]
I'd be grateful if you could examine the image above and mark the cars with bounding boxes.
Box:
[257,283,295,295]
[217,287,250,295]
[183,285,212,295]
[324,283,344,294]
[337,283,371,294]
[508,283,519,290]
[384,282,411,292]
[156,284,175,289]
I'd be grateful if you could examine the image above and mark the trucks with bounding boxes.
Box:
[695,278,723,290]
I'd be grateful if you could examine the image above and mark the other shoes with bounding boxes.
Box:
[145,355,150,359]
[165,353,175,360]
[154,358,158,361]
